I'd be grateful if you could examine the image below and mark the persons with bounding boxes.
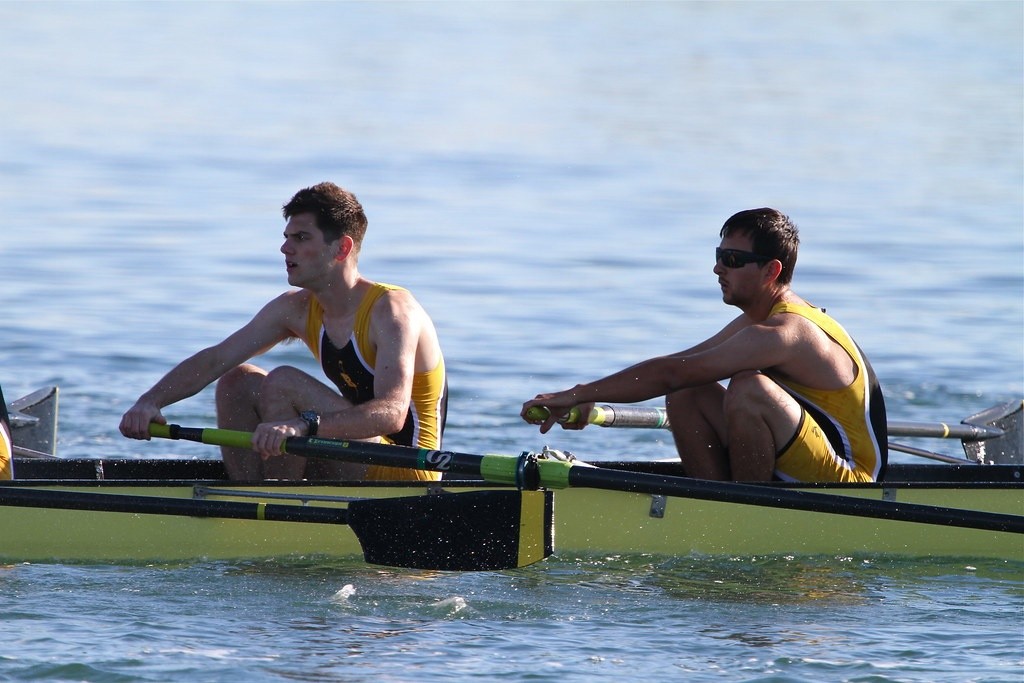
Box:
[0,385,14,479]
[520,208,889,485]
[120,182,449,479]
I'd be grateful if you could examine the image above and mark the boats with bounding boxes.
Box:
[0,458,1023,566]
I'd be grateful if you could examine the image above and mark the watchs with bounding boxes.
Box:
[299,409,320,437]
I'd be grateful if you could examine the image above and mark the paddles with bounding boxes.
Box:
[147,421,1024,533]
[0,386,58,455]
[527,398,1023,465]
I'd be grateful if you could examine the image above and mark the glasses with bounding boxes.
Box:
[716,247,786,269]
